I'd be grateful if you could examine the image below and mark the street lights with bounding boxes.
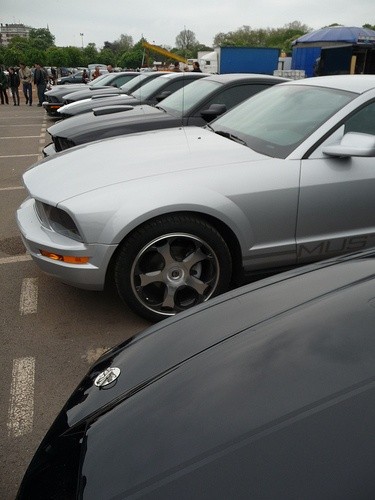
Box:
[80,33,84,57]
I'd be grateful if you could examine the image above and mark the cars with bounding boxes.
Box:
[41,74,296,159]
[43,62,218,126]
[16,246,375,500]
[13,74,375,322]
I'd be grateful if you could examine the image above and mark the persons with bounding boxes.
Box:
[185,62,202,72]
[82,68,89,84]
[107,65,114,73]
[172,61,183,72]
[92,66,102,81]
[8,65,74,87]
[0,67,10,105]
[34,63,46,106]
[19,63,33,106]
[7,67,21,106]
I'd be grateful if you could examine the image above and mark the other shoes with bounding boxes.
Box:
[26,97,28,104]
[14,103,17,106]
[17,104,20,106]
[30,102,32,106]
